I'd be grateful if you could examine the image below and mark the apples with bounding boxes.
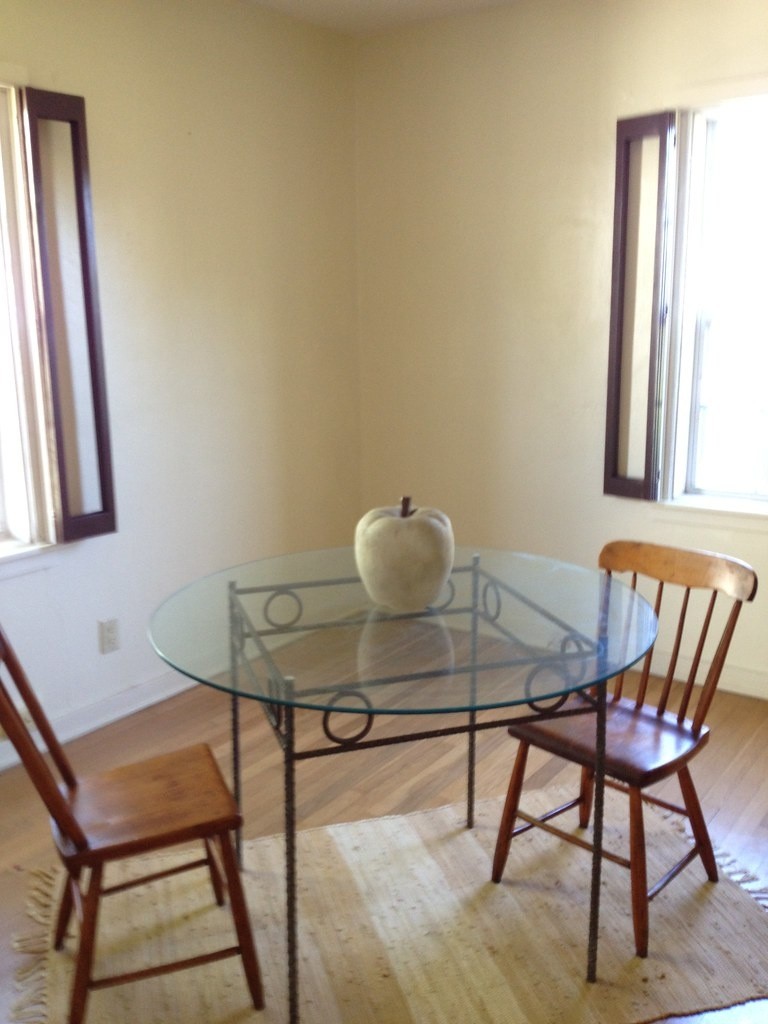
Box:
[353,496,454,611]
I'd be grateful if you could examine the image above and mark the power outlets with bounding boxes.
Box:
[99,617,122,655]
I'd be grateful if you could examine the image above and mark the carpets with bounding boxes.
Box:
[10,771,768,1024]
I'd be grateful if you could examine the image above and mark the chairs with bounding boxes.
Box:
[493,539,759,958]
[0,624,268,1024]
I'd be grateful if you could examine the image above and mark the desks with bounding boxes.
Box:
[144,532,659,1024]
